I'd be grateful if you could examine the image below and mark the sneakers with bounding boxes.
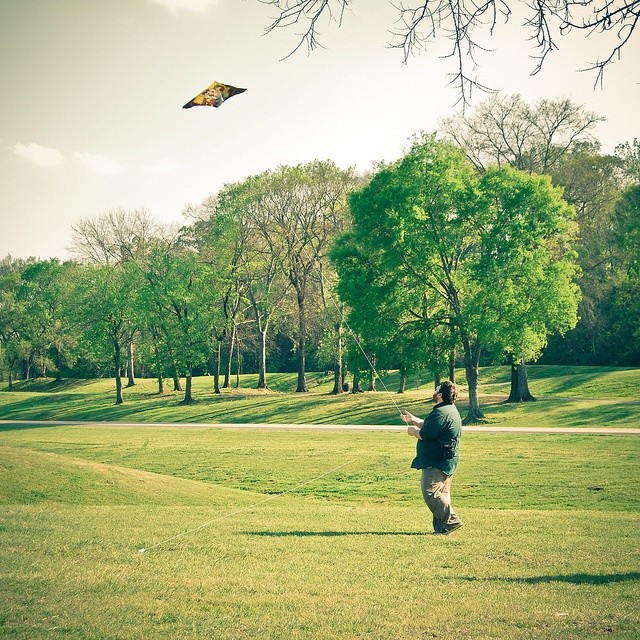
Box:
[442,516,462,535]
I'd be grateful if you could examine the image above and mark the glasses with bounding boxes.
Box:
[436,385,441,392]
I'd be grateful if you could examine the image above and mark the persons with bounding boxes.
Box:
[399,380,465,537]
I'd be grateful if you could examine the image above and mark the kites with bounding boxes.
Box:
[181,80,247,111]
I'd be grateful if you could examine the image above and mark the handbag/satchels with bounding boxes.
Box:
[440,444,454,460]
